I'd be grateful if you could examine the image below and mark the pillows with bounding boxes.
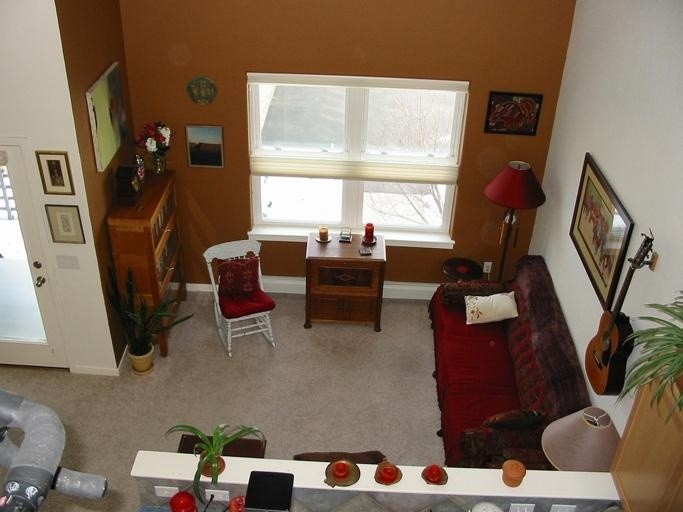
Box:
[215,256,259,296]
[482,409,546,429]
[463,291,519,325]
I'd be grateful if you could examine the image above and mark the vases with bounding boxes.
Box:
[151,153,166,176]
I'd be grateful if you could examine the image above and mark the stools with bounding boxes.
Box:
[442,257,483,284]
[177,434,268,458]
[293,450,388,464]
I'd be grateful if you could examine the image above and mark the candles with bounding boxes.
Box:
[365,223,374,242]
[319,228,328,241]
[333,462,443,484]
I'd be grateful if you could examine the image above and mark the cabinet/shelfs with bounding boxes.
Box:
[304,232,387,332]
[106,169,186,357]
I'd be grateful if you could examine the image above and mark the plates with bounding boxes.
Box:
[315,236,332,243]
[362,236,377,245]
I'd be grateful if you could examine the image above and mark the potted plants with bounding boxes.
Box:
[609,290,683,512]
[106,265,195,376]
[163,423,266,506]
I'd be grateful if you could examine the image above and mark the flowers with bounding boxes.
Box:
[134,120,171,153]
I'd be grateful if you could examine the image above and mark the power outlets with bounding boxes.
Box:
[482,262,493,273]
[205,489,230,501]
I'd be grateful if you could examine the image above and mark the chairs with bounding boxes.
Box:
[202,239,276,358]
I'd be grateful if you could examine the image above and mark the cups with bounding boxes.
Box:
[365,223,374,240]
[320,227,327,239]
[501,459,525,488]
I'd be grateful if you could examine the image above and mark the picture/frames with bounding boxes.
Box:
[569,152,635,311]
[35,150,86,244]
[484,91,544,136]
[185,123,225,169]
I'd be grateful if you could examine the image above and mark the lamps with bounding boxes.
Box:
[541,406,621,472]
[483,160,547,282]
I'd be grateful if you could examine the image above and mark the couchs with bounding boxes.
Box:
[427,255,592,471]
